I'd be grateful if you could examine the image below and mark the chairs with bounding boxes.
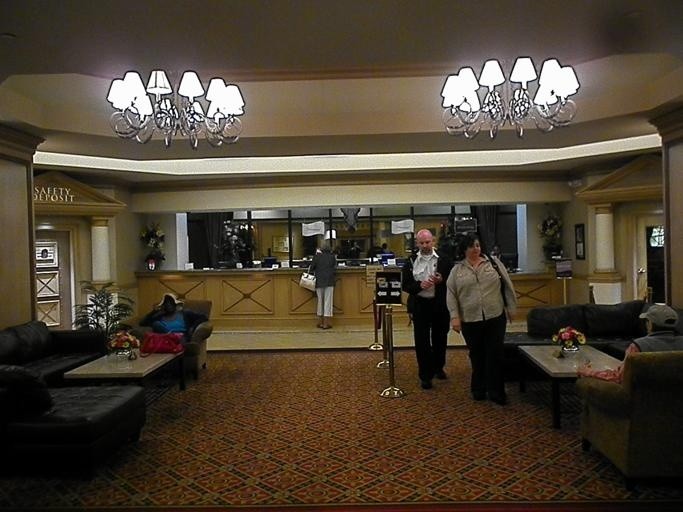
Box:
[576,350,683,491]
[120,299,213,380]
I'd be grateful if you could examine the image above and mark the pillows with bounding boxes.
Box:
[527,304,584,337]
[12,321,52,364]
[0,363,55,418]
[0,335,24,365]
[584,300,647,338]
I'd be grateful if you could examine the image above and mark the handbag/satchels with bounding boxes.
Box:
[299,272,317,293]
[143,331,184,353]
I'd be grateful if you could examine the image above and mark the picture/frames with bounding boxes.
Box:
[574,224,585,260]
[272,236,294,253]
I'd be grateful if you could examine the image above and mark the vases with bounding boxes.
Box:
[542,241,564,260]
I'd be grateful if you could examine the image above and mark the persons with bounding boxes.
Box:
[138,292,207,384]
[401,228,456,390]
[576,301,683,387]
[311,239,336,330]
[446,232,517,401]
[37,249,53,258]
[488,245,508,268]
[339,240,390,258]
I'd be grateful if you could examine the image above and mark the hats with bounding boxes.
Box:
[157,293,184,312]
[639,303,679,328]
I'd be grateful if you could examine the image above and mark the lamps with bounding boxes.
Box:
[440,56,581,140]
[145,258,157,271]
[106,69,246,150]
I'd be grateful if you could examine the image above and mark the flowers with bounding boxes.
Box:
[105,328,141,356]
[138,221,167,258]
[536,213,565,244]
[552,326,586,348]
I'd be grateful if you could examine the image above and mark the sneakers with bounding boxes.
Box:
[317,323,331,329]
[473,390,486,400]
[436,369,445,380]
[422,380,432,389]
[489,390,507,405]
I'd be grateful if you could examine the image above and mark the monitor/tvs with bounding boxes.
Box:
[263,255,277,267]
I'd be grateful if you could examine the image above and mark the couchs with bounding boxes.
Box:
[504,299,683,382]
[0,320,103,388]
[0,384,146,480]
